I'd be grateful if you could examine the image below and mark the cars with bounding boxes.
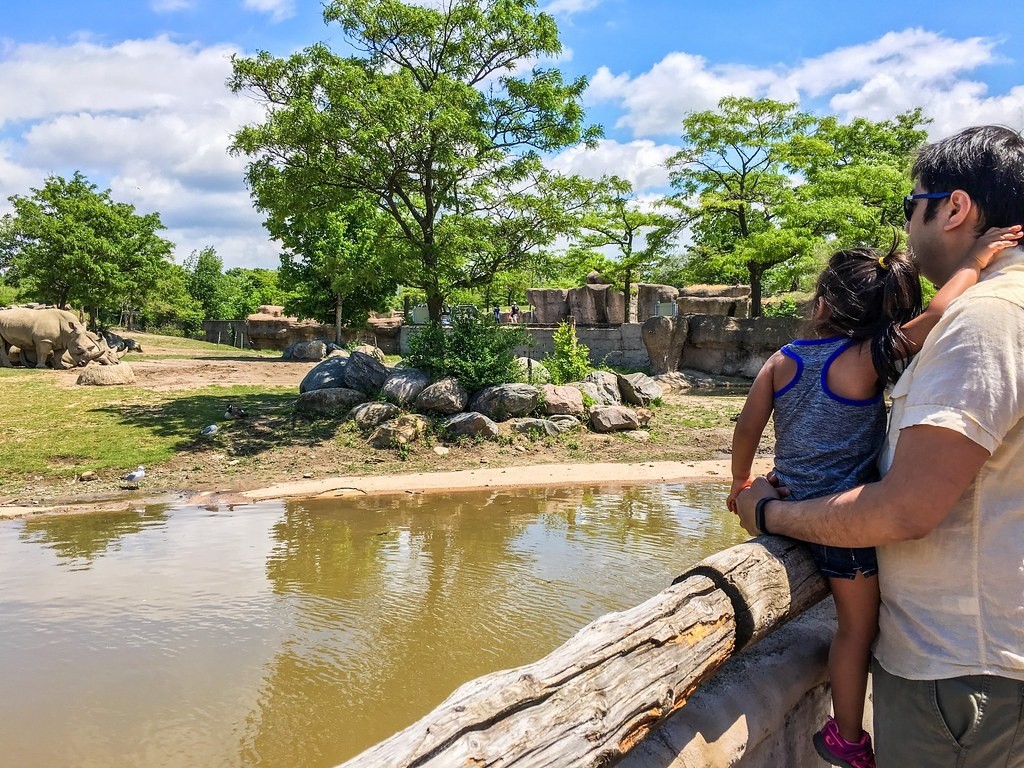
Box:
[450,303,483,327]
[414,304,428,309]
[440,311,451,325]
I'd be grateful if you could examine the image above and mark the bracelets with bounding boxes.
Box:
[754,496,779,535]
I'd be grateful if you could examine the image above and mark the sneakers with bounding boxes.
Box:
[813,714,876,768]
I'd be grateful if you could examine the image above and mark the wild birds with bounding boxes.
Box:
[201,424,222,441]
[124,466,145,489]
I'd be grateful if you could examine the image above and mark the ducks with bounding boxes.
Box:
[224,404,243,419]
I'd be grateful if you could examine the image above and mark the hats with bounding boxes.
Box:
[514,302,516,304]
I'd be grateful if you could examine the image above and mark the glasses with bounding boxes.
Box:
[903,192,950,222]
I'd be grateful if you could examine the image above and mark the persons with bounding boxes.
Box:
[493,305,500,323]
[726,125,1024,768]
[508,302,519,324]
